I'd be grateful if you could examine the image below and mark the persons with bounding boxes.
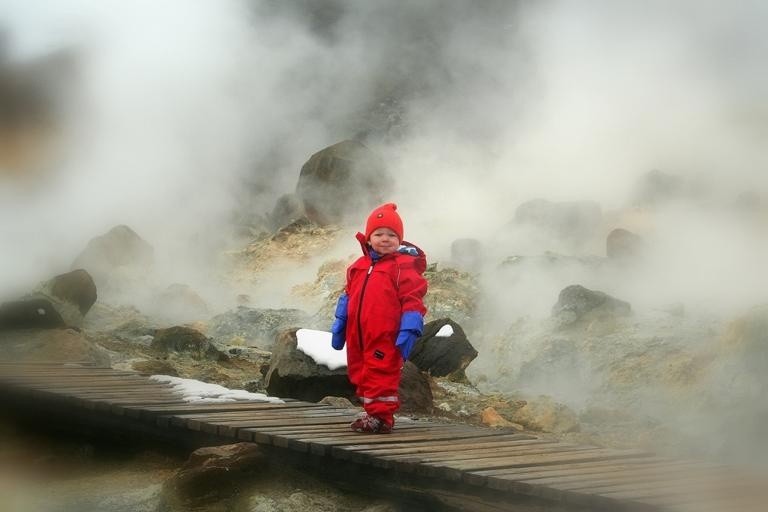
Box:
[330,204,427,434]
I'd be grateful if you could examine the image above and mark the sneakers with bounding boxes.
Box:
[351,413,393,433]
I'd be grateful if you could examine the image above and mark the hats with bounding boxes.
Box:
[365,203,403,246]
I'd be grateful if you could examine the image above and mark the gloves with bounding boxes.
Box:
[396,310,423,362]
[331,295,349,349]
